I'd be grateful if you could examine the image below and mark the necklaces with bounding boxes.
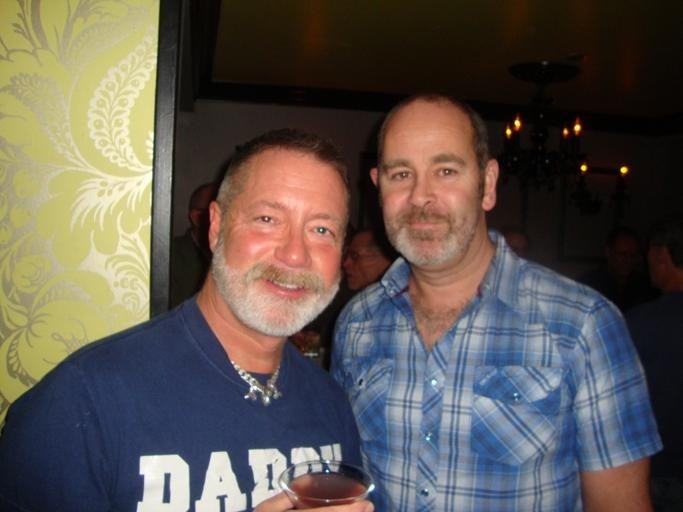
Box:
[231,360,283,407]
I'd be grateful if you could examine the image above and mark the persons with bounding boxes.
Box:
[0,126,384,512]
[328,92,664,512]
[289,222,400,373]
[168,183,219,311]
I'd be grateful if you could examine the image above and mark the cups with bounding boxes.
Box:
[278,458,375,511]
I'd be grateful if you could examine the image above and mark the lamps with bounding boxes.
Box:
[483,59,632,197]
